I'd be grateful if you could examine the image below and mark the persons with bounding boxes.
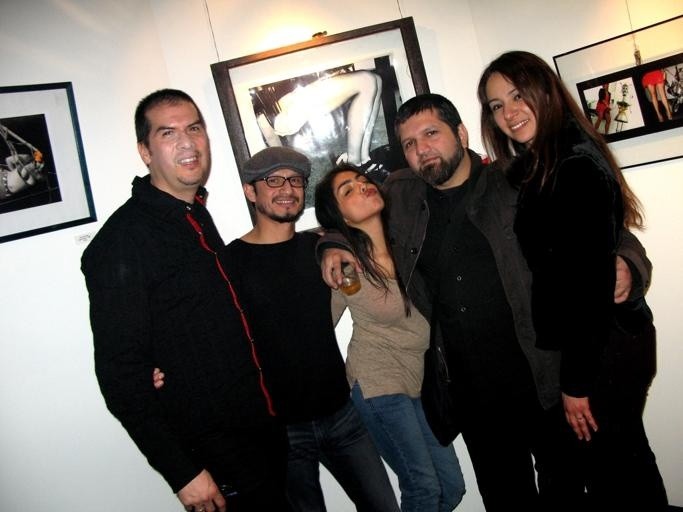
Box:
[315,94,652,512]
[248,72,390,172]
[152,148,401,511]
[308,158,466,511]
[80,88,287,511]
[477,51,668,510]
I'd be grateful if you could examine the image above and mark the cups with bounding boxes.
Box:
[332,265,362,295]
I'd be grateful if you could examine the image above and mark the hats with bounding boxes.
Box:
[242,146,311,184]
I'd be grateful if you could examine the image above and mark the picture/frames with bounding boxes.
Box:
[211,15,449,234]
[0,80,99,245]
[550,14,683,170]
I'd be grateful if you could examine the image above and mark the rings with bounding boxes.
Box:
[576,416,582,421]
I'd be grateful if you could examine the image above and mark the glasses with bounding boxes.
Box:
[252,176,309,189]
